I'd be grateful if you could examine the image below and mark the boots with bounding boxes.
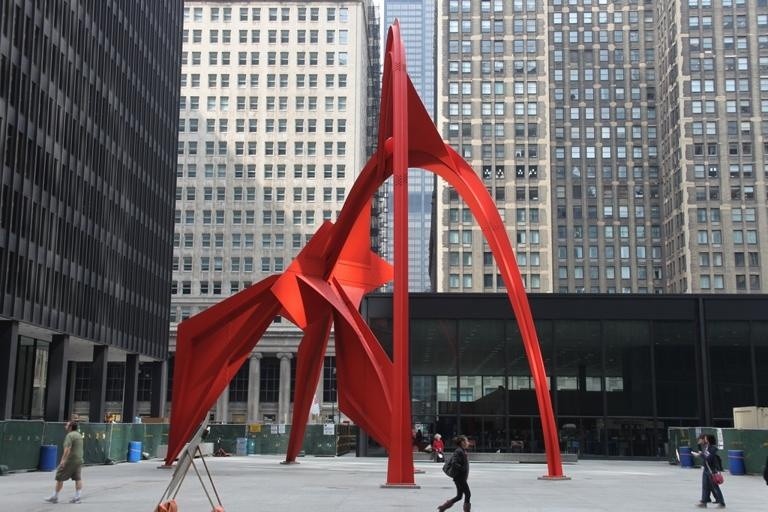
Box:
[437,500,452,512]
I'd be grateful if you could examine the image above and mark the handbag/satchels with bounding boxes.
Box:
[712,472,724,484]
[442,456,454,477]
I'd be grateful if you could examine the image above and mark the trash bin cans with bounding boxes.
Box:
[40,444,57,471]
[247,439,256,454]
[680,447,693,466]
[728,450,745,475]
[128,441,142,463]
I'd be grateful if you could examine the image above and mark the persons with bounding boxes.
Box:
[413,428,422,447]
[435,433,473,512]
[430,434,446,463]
[690,434,722,505]
[42,421,84,506]
[696,435,726,510]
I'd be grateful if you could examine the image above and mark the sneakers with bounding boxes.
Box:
[68,497,81,504]
[44,495,58,504]
